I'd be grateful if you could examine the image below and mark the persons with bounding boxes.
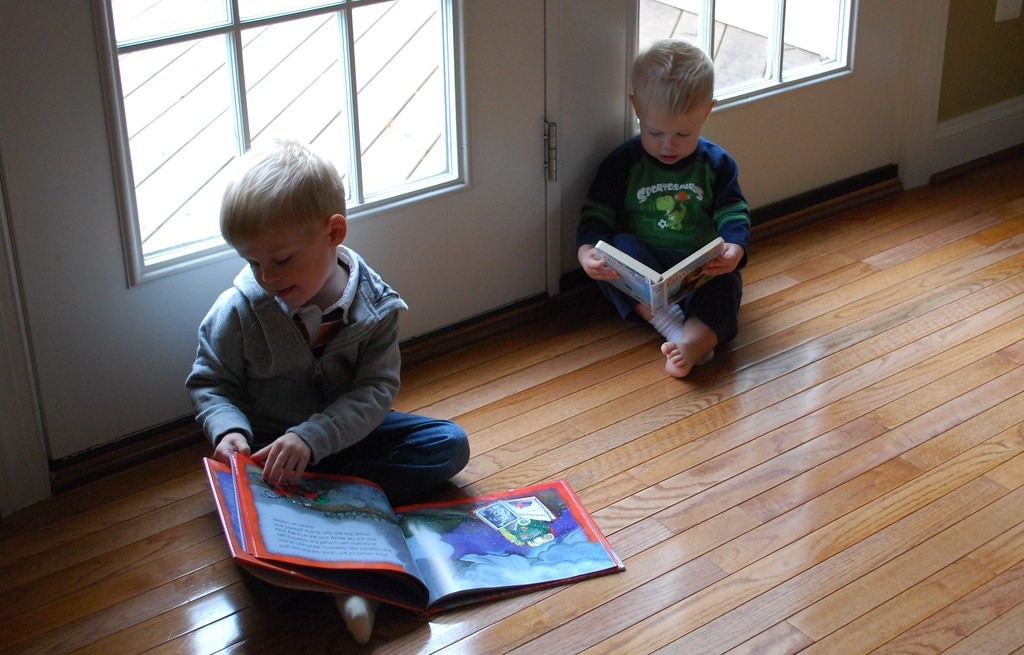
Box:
[576,38,750,380]
[185,138,470,642]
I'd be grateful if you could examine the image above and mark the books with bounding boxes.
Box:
[203,451,625,616]
[594,237,725,316]
